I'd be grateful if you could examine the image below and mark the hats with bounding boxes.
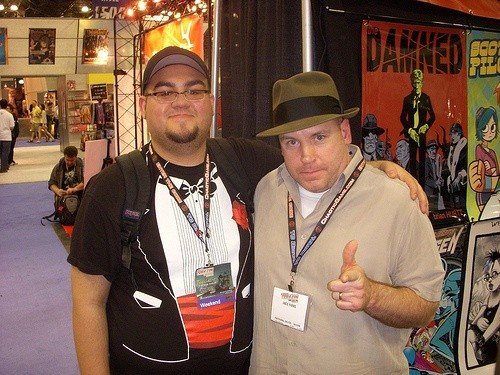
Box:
[143,45,209,93]
[255,70,360,138]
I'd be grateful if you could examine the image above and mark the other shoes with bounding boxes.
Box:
[10,161,15,166]
[27,139,33,143]
[37,140,42,143]
[46,138,50,142]
[0,166,9,173]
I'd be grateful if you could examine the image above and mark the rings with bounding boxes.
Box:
[339,292,342,300]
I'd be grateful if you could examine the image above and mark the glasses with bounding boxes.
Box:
[143,88,211,104]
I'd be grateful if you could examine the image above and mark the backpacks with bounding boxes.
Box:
[54,191,81,226]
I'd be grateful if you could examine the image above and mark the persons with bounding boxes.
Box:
[248,72,446,374]
[0,99,15,173]
[36,104,55,143]
[27,100,49,143]
[5,104,19,165]
[48,146,84,208]
[68,47,430,374]
[29,103,59,141]
